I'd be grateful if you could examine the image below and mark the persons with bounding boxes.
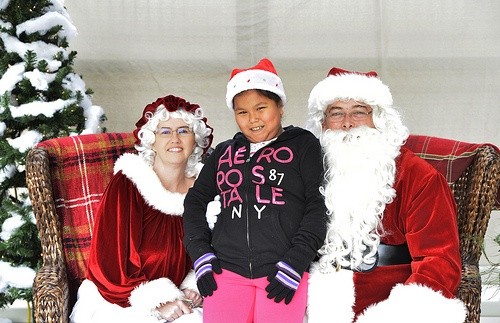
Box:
[183,57,329,323]
[66,95,217,323]
[303,65,469,323]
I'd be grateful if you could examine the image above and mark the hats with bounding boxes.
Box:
[134,94,213,158]
[225,58,287,109]
[308,65,394,110]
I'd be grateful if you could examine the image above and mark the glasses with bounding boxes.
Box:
[152,127,195,137]
[323,108,372,120]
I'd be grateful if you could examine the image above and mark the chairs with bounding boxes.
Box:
[26,132,140,323]
[405,132,499,323]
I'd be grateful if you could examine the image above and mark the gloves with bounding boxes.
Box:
[264,257,306,304]
[193,252,222,296]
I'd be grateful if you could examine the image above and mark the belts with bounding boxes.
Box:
[352,241,413,270]
